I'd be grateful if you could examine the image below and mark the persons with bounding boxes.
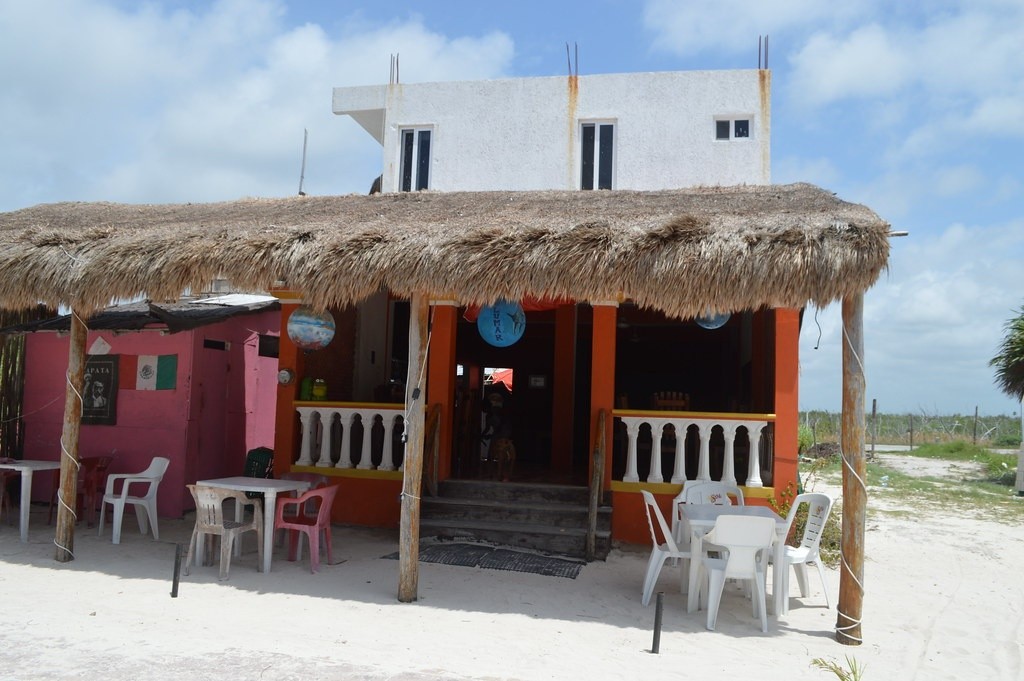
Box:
[482,397,512,465]
[83,379,108,407]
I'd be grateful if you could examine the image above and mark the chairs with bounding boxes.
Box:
[47,457,114,530]
[273,480,342,573]
[99,456,171,544]
[641,479,832,633]
[185,484,263,581]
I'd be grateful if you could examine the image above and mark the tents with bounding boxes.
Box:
[0,184,889,643]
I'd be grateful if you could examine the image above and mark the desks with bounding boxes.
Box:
[196,476,312,575]
[0,459,60,542]
[678,503,790,618]
